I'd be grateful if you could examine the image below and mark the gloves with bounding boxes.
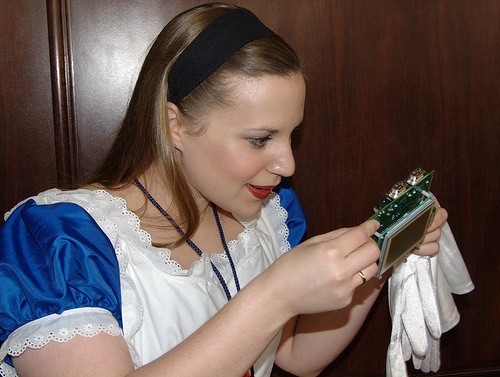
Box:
[384,191,475,377]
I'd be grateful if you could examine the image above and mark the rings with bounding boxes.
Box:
[357,271,367,285]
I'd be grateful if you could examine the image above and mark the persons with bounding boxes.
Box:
[0,3,449,377]
